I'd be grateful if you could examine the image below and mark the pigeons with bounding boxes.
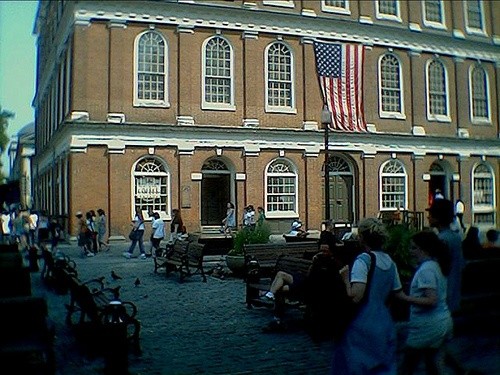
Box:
[134,277,141,286]
[109,270,122,281]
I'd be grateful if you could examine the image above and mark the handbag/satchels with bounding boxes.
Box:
[129,231,136,240]
[327,252,376,310]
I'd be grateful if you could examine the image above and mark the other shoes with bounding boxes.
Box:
[252,297,274,308]
[123,252,132,256]
[146,253,152,257]
[87,253,95,257]
[261,319,285,333]
[140,253,145,257]
[105,244,111,252]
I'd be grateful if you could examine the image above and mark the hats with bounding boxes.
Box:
[291,221,302,229]
[76,212,82,215]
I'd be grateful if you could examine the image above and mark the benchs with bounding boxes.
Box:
[243,241,313,313]
[40,245,142,361]
[153,232,208,284]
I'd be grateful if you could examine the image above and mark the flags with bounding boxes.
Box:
[313,42,368,133]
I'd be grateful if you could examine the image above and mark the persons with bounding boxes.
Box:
[76,209,110,257]
[149,213,164,255]
[398,232,456,375]
[169,208,189,241]
[0,206,62,272]
[289,220,303,235]
[480,229,500,248]
[456,200,467,232]
[222,201,236,239]
[330,220,409,375]
[242,204,267,231]
[428,200,477,375]
[461,224,481,260]
[247,232,340,333]
[428,185,444,206]
[122,207,146,258]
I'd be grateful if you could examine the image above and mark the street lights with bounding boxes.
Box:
[319,102,332,229]
